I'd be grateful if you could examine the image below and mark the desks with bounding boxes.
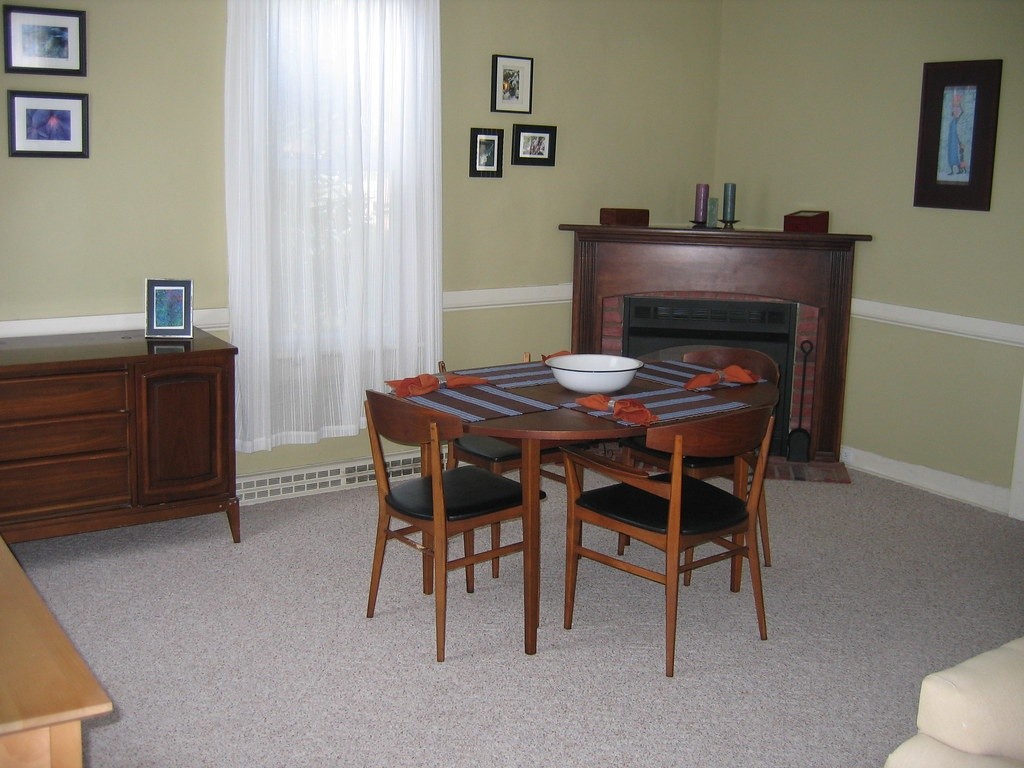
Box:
[0,532,113,768]
[388,361,779,655]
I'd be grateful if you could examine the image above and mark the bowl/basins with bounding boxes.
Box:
[545,353,644,395]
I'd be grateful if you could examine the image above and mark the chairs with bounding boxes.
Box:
[617,348,780,586]
[365,390,547,663]
[436,353,583,578]
[558,406,778,678]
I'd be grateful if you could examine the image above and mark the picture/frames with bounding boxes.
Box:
[469,128,504,177]
[913,58,1003,211]
[2,5,86,77]
[7,90,90,158]
[144,279,194,339]
[512,124,556,166]
[491,54,533,114]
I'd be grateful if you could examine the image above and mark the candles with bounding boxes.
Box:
[695,184,709,221]
[722,183,736,221]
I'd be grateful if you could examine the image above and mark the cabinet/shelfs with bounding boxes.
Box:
[0,325,241,544]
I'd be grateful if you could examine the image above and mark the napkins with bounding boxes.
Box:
[543,351,570,367]
[685,365,761,390]
[384,373,488,397]
[574,394,660,427]
